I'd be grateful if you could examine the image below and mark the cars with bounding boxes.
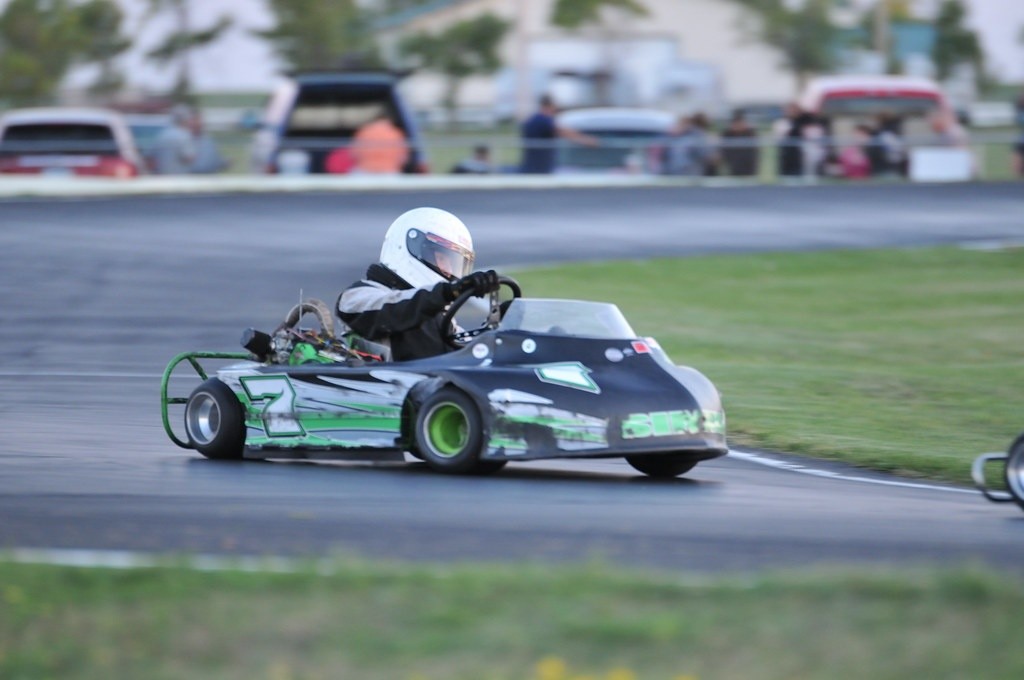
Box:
[3,108,151,178]
[794,80,962,178]
[257,66,426,175]
[534,107,703,176]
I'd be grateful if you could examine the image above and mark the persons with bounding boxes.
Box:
[335,206,514,362]
[148,100,232,175]
[349,115,411,172]
[519,95,601,175]
[623,107,761,176]
[769,97,982,181]
[455,144,495,175]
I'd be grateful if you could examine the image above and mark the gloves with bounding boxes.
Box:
[449,270,499,299]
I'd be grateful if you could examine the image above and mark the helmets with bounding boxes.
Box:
[380,207,475,292]
